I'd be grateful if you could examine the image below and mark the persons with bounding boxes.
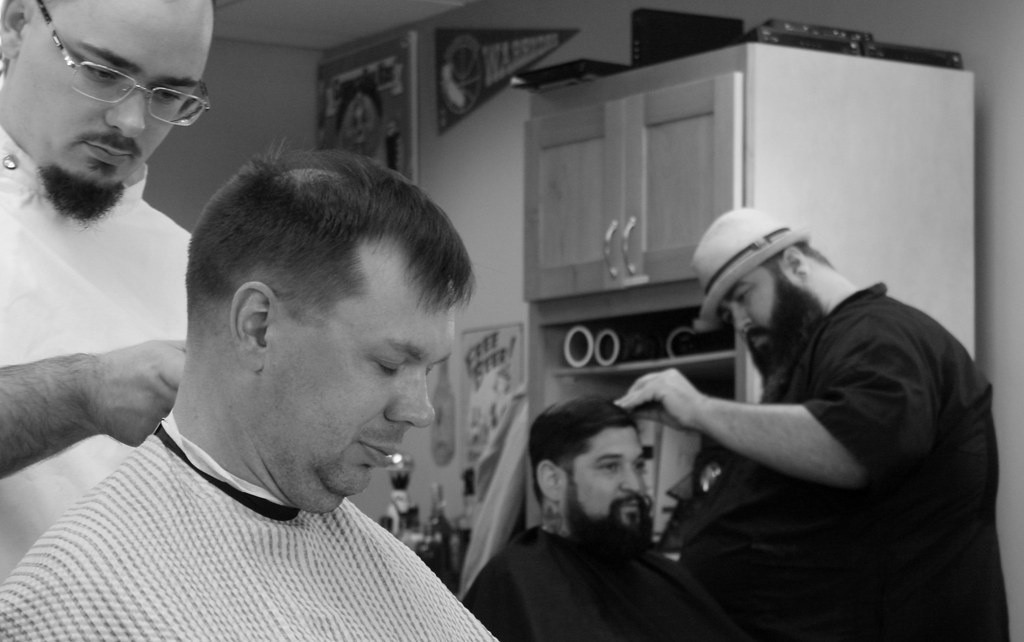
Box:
[0,149,501,642]
[612,204,1011,642]
[460,394,757,640]
[1,0,217,579]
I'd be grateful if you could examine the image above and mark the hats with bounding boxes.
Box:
[693,208,809,333]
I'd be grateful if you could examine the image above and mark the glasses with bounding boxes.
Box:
[34,0,210,127]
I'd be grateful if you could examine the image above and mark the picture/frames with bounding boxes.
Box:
[317,29,419,184]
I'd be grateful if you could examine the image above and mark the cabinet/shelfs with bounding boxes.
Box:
[526,69,977,301]
